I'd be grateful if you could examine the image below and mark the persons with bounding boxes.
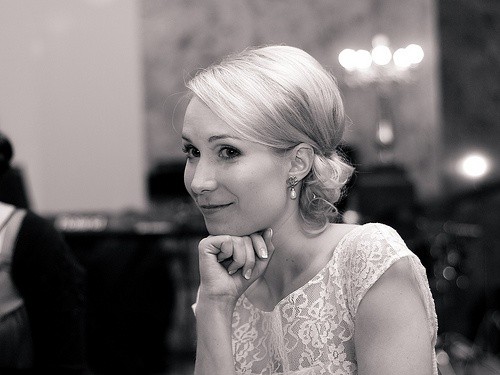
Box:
[0,132,212,375]
[180,46,438,375]
